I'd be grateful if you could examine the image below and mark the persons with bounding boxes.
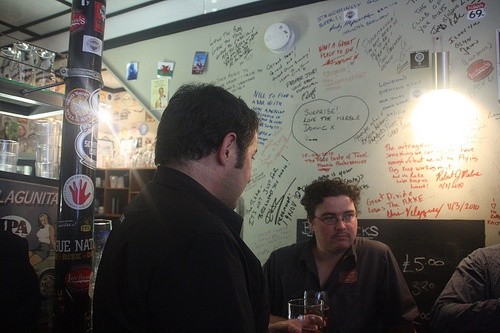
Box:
[260,177,423,333]
[0,229,41,333]
[93,82,270,333]
[429,244,500,333]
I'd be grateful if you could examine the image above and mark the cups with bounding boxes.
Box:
[34,120,61,179]
[288,299,324,333]
[0,140,20,174]
[304,290,326,333]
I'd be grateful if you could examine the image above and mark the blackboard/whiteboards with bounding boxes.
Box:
[295,218,487,333]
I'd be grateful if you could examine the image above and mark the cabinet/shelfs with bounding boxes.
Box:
[93,167,158,217]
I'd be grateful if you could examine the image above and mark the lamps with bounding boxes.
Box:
[430,50,451,90]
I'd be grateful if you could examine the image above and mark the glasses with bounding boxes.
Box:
[314,214,357,225]
